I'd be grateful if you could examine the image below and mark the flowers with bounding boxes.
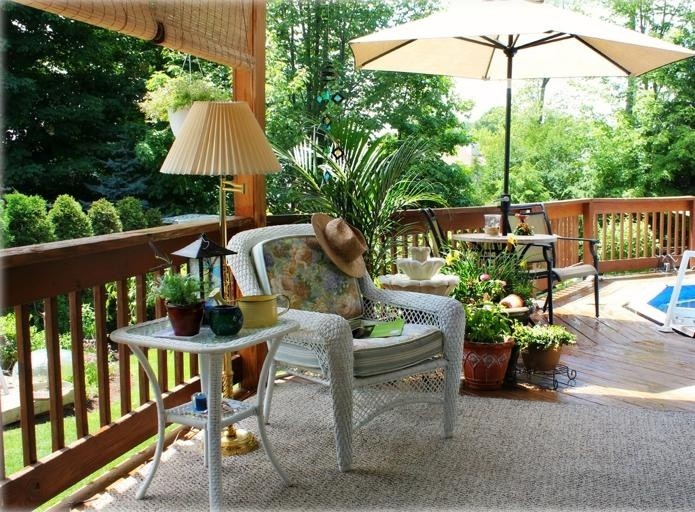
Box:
[471,273,507,302]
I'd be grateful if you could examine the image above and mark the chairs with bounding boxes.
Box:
[224,222,465,470]
[497,203,601,320]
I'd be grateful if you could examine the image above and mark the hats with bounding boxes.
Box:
[311,212,369,279]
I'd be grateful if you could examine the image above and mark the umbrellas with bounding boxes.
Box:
[348,0,695,251]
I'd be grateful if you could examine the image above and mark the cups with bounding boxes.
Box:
[483,213,502,235]
[190,392,208,415]
[209,305,243,336]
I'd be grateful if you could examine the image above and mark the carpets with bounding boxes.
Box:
[71,372,695,512]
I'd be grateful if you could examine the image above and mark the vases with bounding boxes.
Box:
[483,300,530,325]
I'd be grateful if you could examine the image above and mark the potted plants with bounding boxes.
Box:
[510,318,578,371]
[135,68,232,139]
[463,303,515,391]
[150,257,213,337]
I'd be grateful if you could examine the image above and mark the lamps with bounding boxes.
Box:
[158,101,285,456]
